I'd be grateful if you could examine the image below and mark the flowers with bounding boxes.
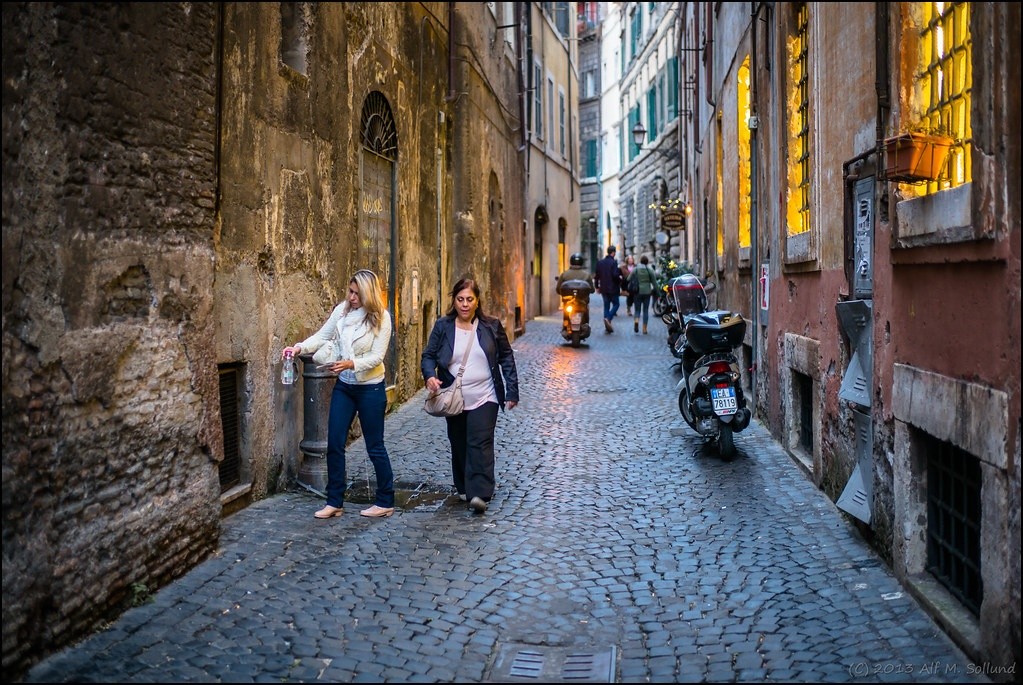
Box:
[659,255,694,294]
[647,198,687,211]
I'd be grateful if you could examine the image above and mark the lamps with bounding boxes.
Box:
[632,121,678,158]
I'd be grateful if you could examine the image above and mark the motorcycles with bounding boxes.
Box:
[654,274,709,357]
[662,295,752,466]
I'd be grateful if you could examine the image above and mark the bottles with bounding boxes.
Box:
[282,351,294,385]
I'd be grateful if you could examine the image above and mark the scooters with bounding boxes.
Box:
[557,272,593,346]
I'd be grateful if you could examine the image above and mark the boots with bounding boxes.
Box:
[626,306,633,316]
[634,317,639,333]
[643,324,648,334]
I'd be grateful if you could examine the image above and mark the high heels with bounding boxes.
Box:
[360,505,394,517]
[315,505,343,518]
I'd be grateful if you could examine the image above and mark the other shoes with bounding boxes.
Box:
[613,313,618,315]
[460,494,466,501]
[603,318,614,334]
[470,496,486,514]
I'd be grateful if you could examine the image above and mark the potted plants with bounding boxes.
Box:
[886,119,959,185]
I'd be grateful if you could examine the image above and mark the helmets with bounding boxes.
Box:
[570,253,584,266]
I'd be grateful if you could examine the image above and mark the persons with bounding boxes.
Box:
[420,278,520,510]
[281,270,394,518]
[595,246,622,333]
[622,255,637,316]
[556,254,595,337]
[627,256,660,333]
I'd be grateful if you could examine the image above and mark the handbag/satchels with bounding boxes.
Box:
[312,309,344,368]
[424,375,464,417]
[628,267,639,292]
[650,283,656,296]
